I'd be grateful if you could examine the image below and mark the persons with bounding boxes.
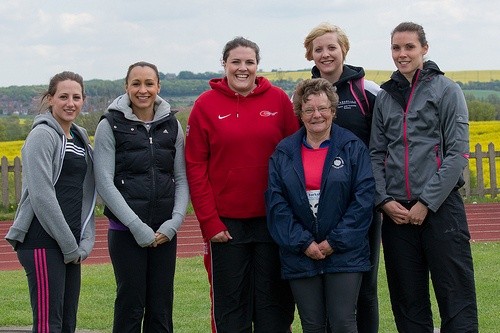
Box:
[93,62,189,333]
[4,71,98,333]
[304,20,388,333]
[369,22,480,333]
[267,78,376,333]
[185,37,301,333]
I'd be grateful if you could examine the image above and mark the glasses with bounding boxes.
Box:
[301,105,332,116]
